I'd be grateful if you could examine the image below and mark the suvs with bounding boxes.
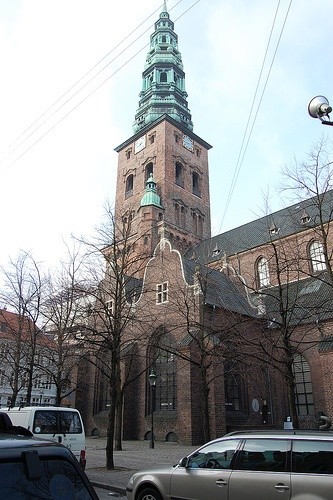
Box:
[127,430,332,500]
[0,410,101,500]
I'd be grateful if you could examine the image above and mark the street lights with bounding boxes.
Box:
[38,384,45,407]
[149,370,157,449]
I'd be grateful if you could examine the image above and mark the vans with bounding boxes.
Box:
[0,402,87,475]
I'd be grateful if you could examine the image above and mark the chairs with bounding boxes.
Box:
[248,451,285,471]
